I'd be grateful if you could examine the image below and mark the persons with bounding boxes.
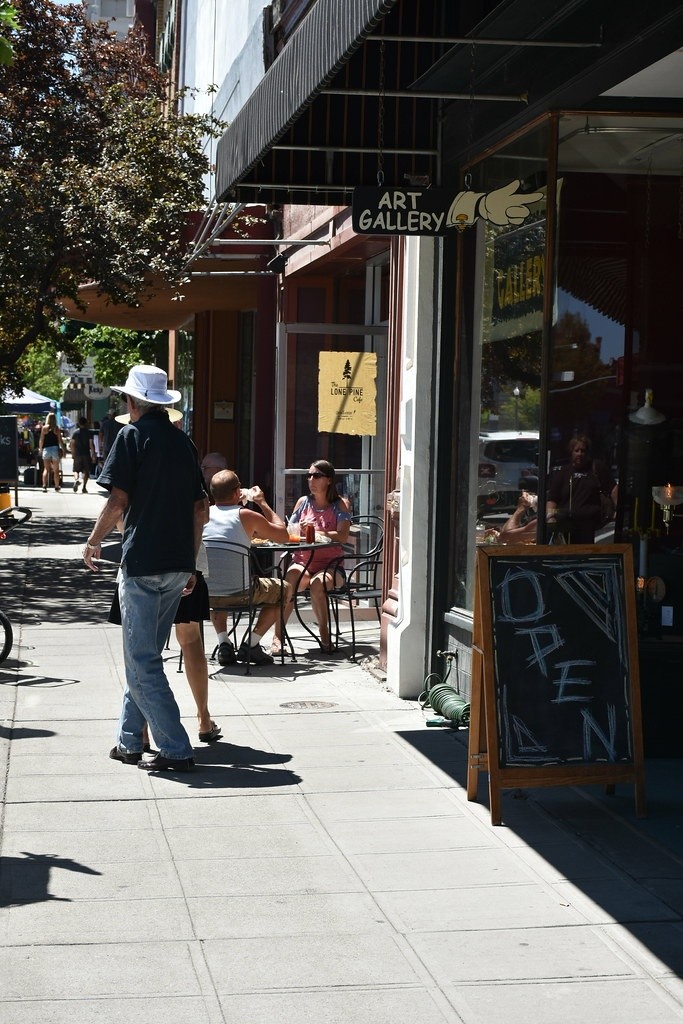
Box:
[546,436,618,544]
[273,459,352,656]
[501,496,538,543]
[89,421,104,478]
[98,408,125,460]
[70,417,96,493]
[82,364,202,772]
[202,452,228,505]
[39,413,66,493]
[114,407,221,748]
[202,469,292,663]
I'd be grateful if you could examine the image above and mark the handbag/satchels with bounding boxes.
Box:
[595,474,614,525]
[59,449,63,458]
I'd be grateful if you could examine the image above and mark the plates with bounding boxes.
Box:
[299,537,307,541]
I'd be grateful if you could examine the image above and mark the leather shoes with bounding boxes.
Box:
[110,746,142,764]
[138,753,195,769]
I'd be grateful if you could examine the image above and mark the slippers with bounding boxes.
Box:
[199,721,221,741]
[143,743,151,752]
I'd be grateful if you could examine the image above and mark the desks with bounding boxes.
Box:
[209,540,341,661]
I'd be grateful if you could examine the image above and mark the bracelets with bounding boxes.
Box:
[87,541,100,550]
[546,513,555,519]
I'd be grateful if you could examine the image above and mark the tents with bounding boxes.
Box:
[0,387,57,414]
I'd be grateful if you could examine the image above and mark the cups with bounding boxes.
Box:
[288,523,300,542]
[476,525,485,543]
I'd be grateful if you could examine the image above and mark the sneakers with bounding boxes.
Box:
[237,643,274,663]
[218,642,236,664]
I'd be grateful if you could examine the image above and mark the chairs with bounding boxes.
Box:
[177,540,287,675]
[277,515,384,664]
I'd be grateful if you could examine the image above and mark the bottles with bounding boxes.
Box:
[306,523,315,544]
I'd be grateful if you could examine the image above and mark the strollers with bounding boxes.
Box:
[0,506,31,661]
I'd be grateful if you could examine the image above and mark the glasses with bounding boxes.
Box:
[305,472,329,480]
[201,466,221,469]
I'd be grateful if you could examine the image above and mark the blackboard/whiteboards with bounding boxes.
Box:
[469,543,643,785]
[0,415,18,483]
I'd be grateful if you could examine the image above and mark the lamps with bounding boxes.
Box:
[628,389,666,426]
[651,481,683,536]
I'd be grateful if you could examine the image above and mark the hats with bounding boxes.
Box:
[114,407,184,425]
[109,365,181,404]
[105,409,117,414]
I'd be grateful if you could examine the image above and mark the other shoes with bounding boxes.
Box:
[321,642,335,652]
[74,480,79,492]
[43,489,47,492]
[82,489,87,493]
[55,486,61,491]
[272,643,287,655]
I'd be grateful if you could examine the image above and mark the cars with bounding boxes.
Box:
[476,429,543,519]
